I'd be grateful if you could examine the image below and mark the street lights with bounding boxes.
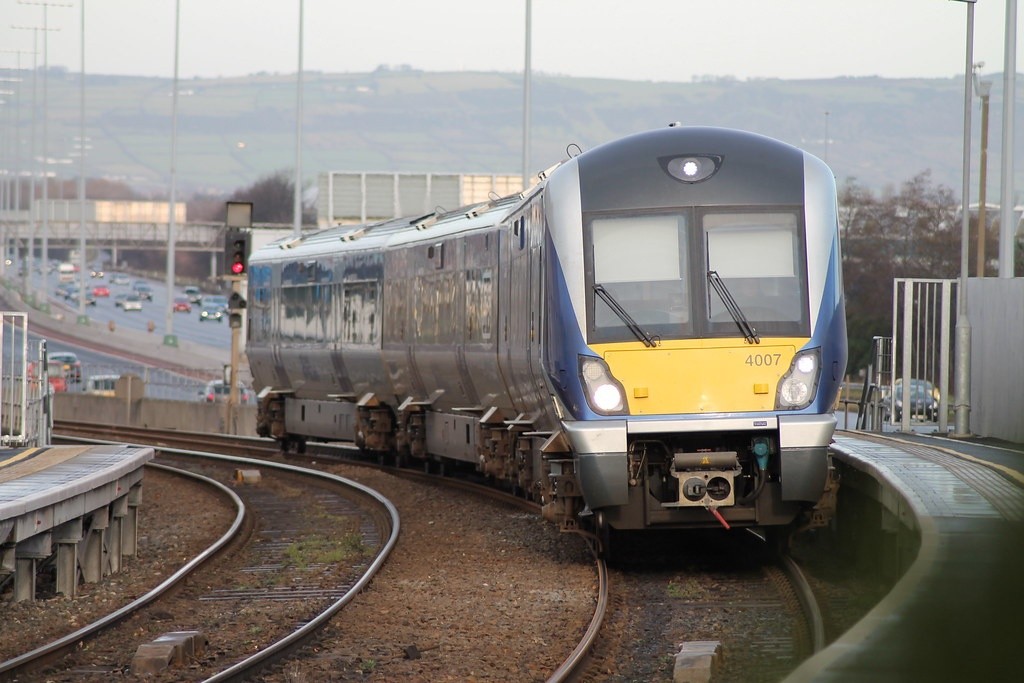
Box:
[971,63,992,276]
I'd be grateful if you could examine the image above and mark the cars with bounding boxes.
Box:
[199,306,222,321]
[198,379,249,404]
[81,375,119,397]
[882,386,939,423]
[46,352,81,382]
[18,258,153,311]
[893,378,941,404]
[174,297,190,313]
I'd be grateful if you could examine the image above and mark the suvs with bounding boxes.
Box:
[183,287,202,305]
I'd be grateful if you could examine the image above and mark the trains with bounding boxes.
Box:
[245,123,849,557]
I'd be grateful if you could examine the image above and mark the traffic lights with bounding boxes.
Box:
[227,231,250,276]
